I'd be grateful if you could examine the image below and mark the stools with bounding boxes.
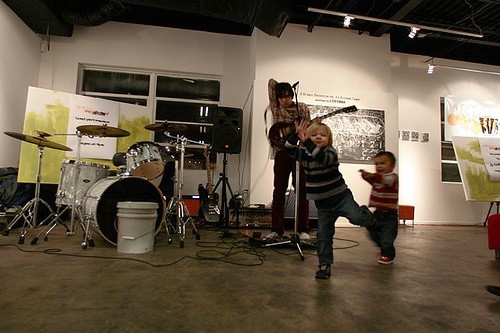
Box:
[488,214,500,259]
[399,205,414,228]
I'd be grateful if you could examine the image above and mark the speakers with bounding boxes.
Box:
[212,107,242,155]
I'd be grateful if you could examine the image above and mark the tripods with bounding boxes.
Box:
[3,133,95,247]
[165,130,200,248]
[261,160,317,260]
[213,153,233,229]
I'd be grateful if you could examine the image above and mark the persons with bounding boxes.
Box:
[285,119,385,280]
[358,152,398,265]
[269,79,312,240]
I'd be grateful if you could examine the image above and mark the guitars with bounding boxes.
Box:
[268,105,358,152]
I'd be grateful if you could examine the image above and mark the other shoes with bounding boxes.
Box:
[360,206,377,231]
[299,232,311,243]
[261,232,283,243]
[377,256,393,264]
[314,263,331,279]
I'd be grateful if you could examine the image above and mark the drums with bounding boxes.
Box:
[80,174,168,246]
[124,140,179,196]
[54,159,109,209]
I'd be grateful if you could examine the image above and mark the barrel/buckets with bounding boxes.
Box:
[114,201,158,254]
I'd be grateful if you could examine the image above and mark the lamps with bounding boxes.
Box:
[408,26,420,38]
[427,60,436,74]
[343,16,355,27]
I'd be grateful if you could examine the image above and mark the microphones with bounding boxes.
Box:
[292,82,299,88]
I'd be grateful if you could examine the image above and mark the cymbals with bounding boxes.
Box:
[75,124,130,138]
[2,130,73,152]
[144,121,188,132]
